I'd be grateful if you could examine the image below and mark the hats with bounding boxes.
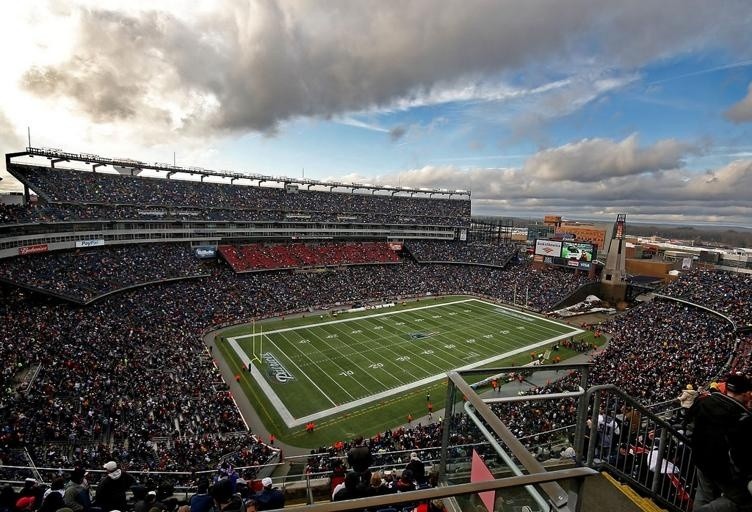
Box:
[262,477,272,487]
[102,461,117,470]
[726,375,751,394]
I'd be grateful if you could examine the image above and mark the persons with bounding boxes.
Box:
[1,168,252,512]
[320,314,323,320]
[253,184,598,318]
[253,384,554,512]
[492,350,544,385]
[554,269,752,512]
[281,315,285,320]
